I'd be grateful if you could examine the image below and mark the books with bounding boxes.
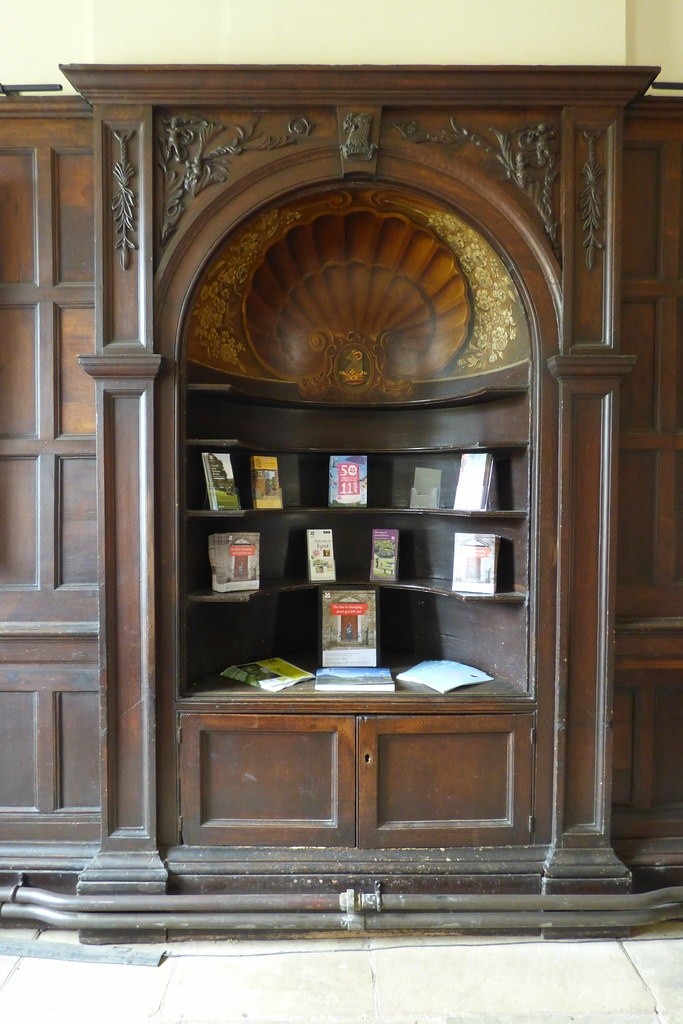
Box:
[306,529,336,581]
[452,532,500,593]
[207,532,260,592]
[249,456,283,509]
[315,667,395,691]
[370,529,399,583]
[220,659,316,693]
[201,453,241,510]
[319,585,380,668]
[329,455,367,508]
[453,453,493,511]
[396,659,496,693]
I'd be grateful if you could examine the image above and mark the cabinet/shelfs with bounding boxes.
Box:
[180,382,533,705]
